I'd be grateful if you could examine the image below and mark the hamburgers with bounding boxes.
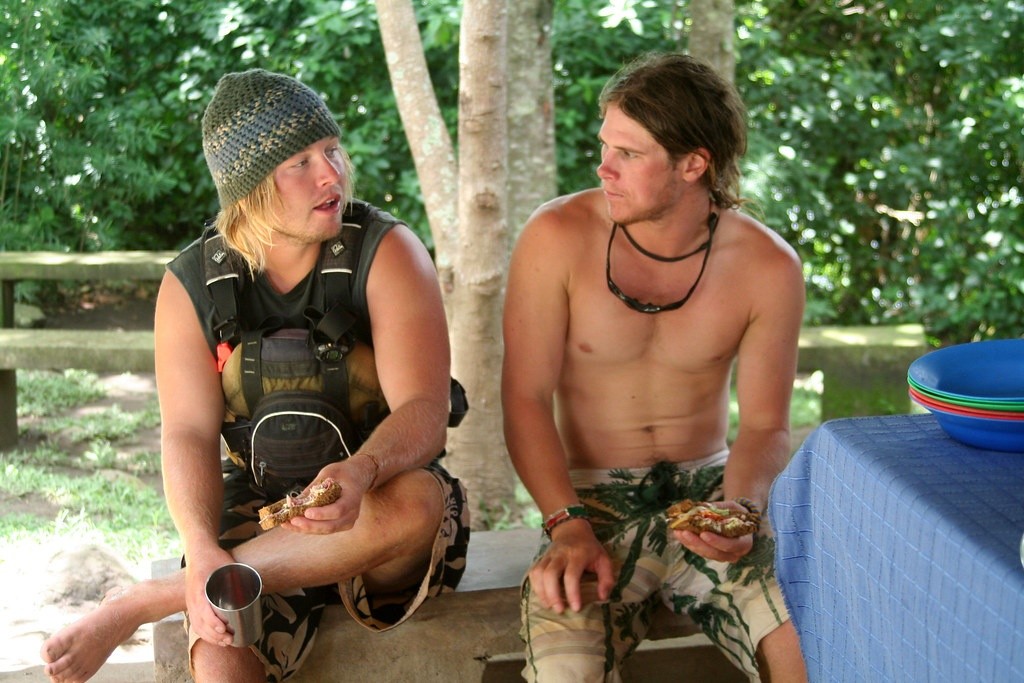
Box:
[258,478,342,530]
[665,500,758,538]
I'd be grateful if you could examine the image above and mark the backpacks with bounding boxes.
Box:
[203,198,468,512]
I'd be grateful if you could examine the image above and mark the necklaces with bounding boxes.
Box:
[618,210,716,262]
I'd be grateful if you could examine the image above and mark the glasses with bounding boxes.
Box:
[605,212,718,313]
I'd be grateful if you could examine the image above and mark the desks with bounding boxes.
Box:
[765,412,1024,682]
[0,248,180,452]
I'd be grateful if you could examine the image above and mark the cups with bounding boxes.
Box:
[204,562,263,649]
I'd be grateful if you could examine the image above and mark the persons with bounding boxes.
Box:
[44,67,473,683]
[501,56,807,682]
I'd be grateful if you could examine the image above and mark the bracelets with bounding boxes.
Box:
[348,451,380,495]
[540,503,593,542]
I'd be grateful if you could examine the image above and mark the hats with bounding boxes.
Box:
[202,69,342,207]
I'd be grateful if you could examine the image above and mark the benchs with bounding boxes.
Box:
[150,521,750,682]
[0,327,156,371]
[794,322,927,424]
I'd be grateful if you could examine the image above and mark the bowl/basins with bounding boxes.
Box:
[907,338,1024,452]
